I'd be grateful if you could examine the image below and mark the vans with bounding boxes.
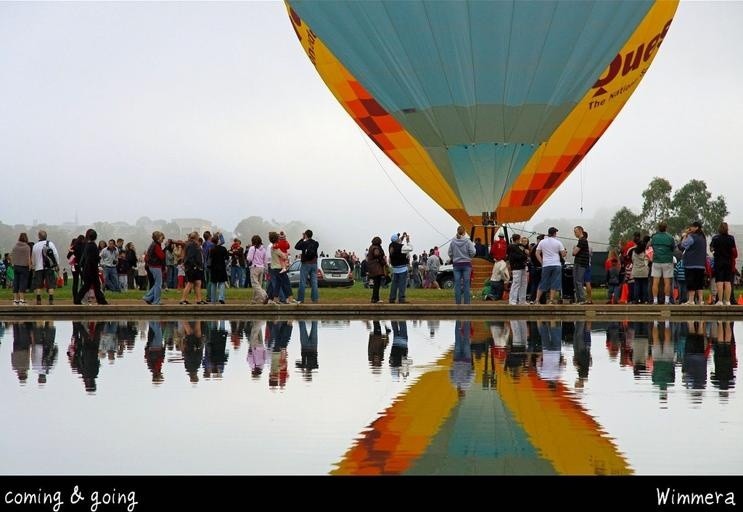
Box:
[286,257,354,287]
[584,251,621,288]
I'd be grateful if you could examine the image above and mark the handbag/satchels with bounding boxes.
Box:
[42,241,57,268]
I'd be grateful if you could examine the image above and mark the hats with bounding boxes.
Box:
[280,232,286,240]
[549,228,558,234]
[391,233,400,242]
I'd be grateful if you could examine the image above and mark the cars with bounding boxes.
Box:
[435,265,453,288]
[528,263,574,298]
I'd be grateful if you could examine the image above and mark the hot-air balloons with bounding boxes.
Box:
[284,1,678,290]
[327,319,635,476]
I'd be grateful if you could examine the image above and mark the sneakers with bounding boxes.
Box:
[141,296,225,304]
[252,297,318,305]
[608,297,738,305]
[372,298,409,304]
[13,297,109,305]
[484,293,592,305]
[280,268,286,273]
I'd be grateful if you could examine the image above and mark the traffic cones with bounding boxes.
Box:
[606,292,618,305]
[705,294,714,304]
[672,289,679,303]
[618,283,630,304]
[736,294,743,305]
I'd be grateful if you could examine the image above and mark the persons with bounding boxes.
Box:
[367,237,391,304]
[0,319,319,394]
[389,232,412,304]
[388,321,413,380]
[320,249,362,281]
[408,225,593,305]
[605,222,743,305]
[603,319,739,406]
[367,319,390,373]
[450,321,593,401]
[294,230,319,304]
[0,229,296,305]
[426,321,439,336]
